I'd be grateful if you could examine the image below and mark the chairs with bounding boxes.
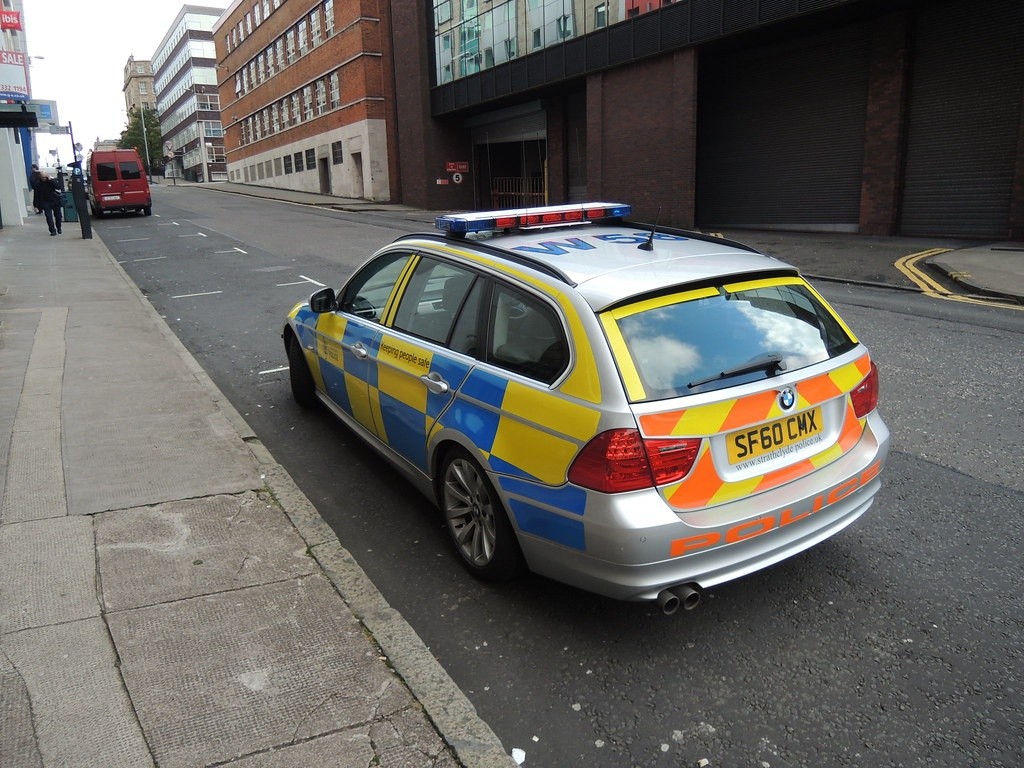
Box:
[428,275,551,364]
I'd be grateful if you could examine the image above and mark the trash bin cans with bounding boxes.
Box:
[62,190,79,223]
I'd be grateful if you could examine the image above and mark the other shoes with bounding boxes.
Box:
[50,230,61,236]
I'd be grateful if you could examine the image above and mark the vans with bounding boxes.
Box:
[86,145,153,221]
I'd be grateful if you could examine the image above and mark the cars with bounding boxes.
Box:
[284,195,891,615]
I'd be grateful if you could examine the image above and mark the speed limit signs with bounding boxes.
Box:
[453,172,462,183]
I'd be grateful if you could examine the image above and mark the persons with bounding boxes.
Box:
[30,169,64,237]
[29,164,46,214]
